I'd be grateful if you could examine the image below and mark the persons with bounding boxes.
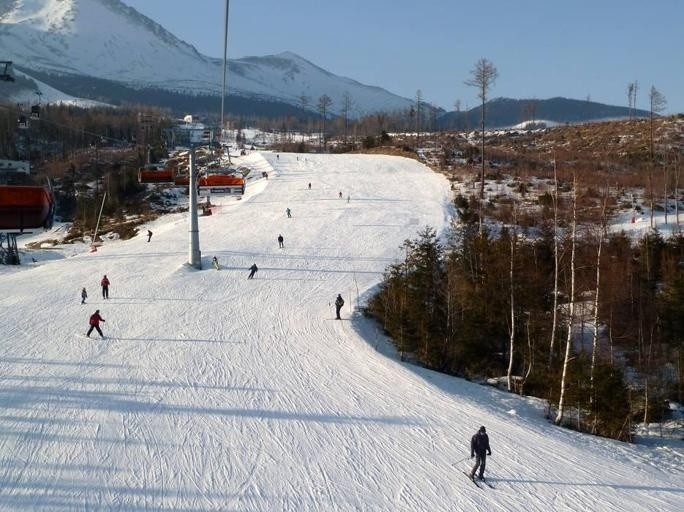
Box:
[333,293,344,320]
[85,308,106,340]
[277,233,283,249]
[146,229,152,242]
[100,274,110,300]
[246,263,258,279]
[471,424,491,482]
[284,207,290,218]
[80,287,87,305]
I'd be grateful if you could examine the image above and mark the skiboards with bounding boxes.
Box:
[463,471,494,489]
